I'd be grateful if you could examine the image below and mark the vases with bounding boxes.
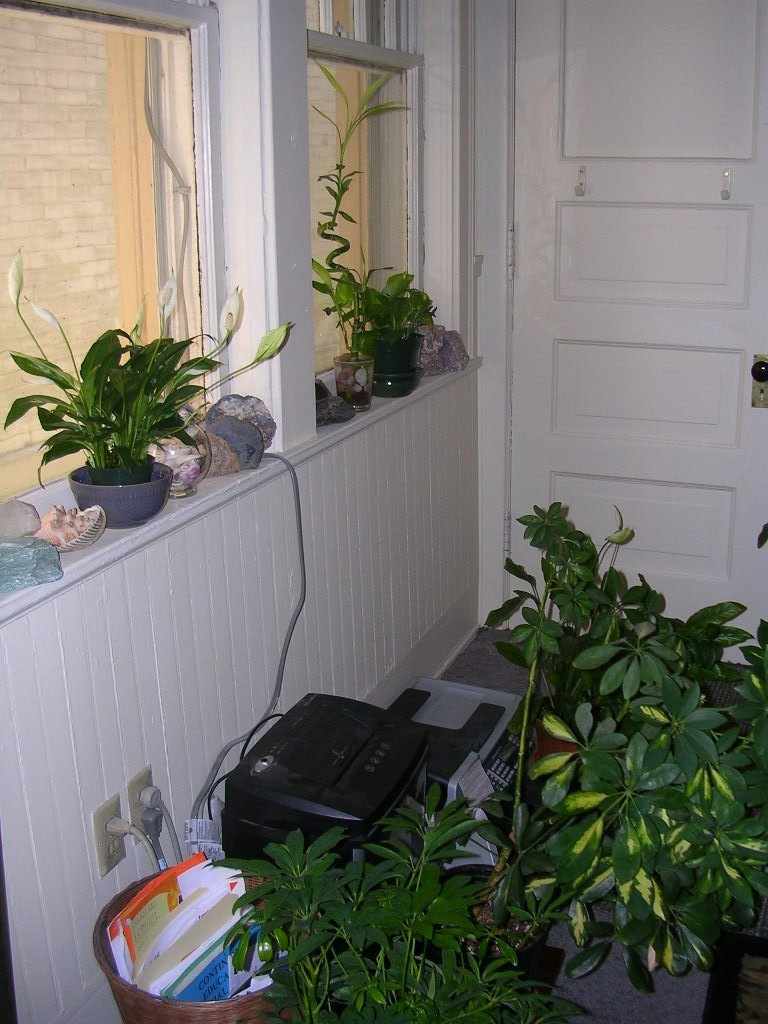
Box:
[87,456,163,486]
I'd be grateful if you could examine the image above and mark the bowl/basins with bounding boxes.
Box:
[68,462,173,528]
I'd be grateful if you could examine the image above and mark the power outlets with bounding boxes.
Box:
[92,794,126,879]
[128,765,157,846]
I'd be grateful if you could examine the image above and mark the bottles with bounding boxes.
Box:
[148,409,212,500]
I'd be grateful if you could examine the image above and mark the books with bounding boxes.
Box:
[105,852,292,1001]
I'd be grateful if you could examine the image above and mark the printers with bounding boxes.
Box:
[221,693,434,883]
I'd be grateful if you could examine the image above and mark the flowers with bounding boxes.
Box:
[1,249,294,491]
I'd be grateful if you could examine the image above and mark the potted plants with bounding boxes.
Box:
[312,61,415,410]
[201,502,768,1023]
[312,271,441,396]
